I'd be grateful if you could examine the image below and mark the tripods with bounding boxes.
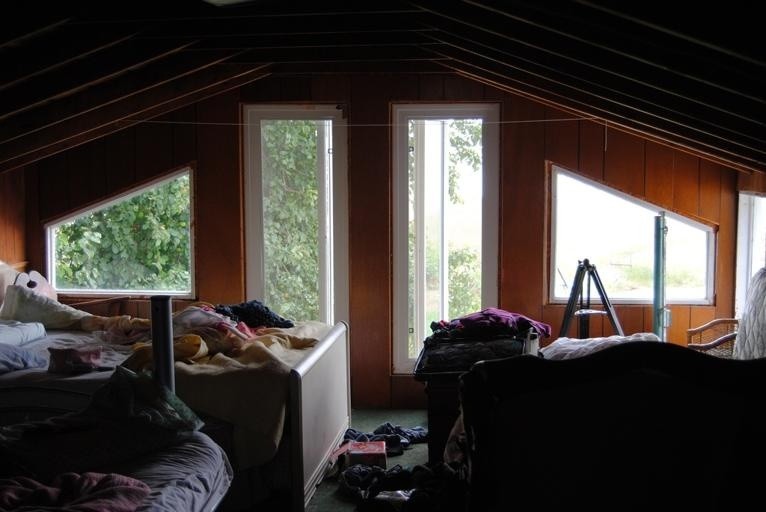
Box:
[561,257,625,338]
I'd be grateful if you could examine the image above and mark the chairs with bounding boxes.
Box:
[685,318,745,358]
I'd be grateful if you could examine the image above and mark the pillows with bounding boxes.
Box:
[0,317,51,343]
[30,364,208,469]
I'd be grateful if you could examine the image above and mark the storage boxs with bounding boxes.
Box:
[348,439,388,471]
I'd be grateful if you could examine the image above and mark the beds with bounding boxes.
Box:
[0,311,353,512]
[0,411,235,512]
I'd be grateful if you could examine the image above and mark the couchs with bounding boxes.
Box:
[442,341,764,510]
